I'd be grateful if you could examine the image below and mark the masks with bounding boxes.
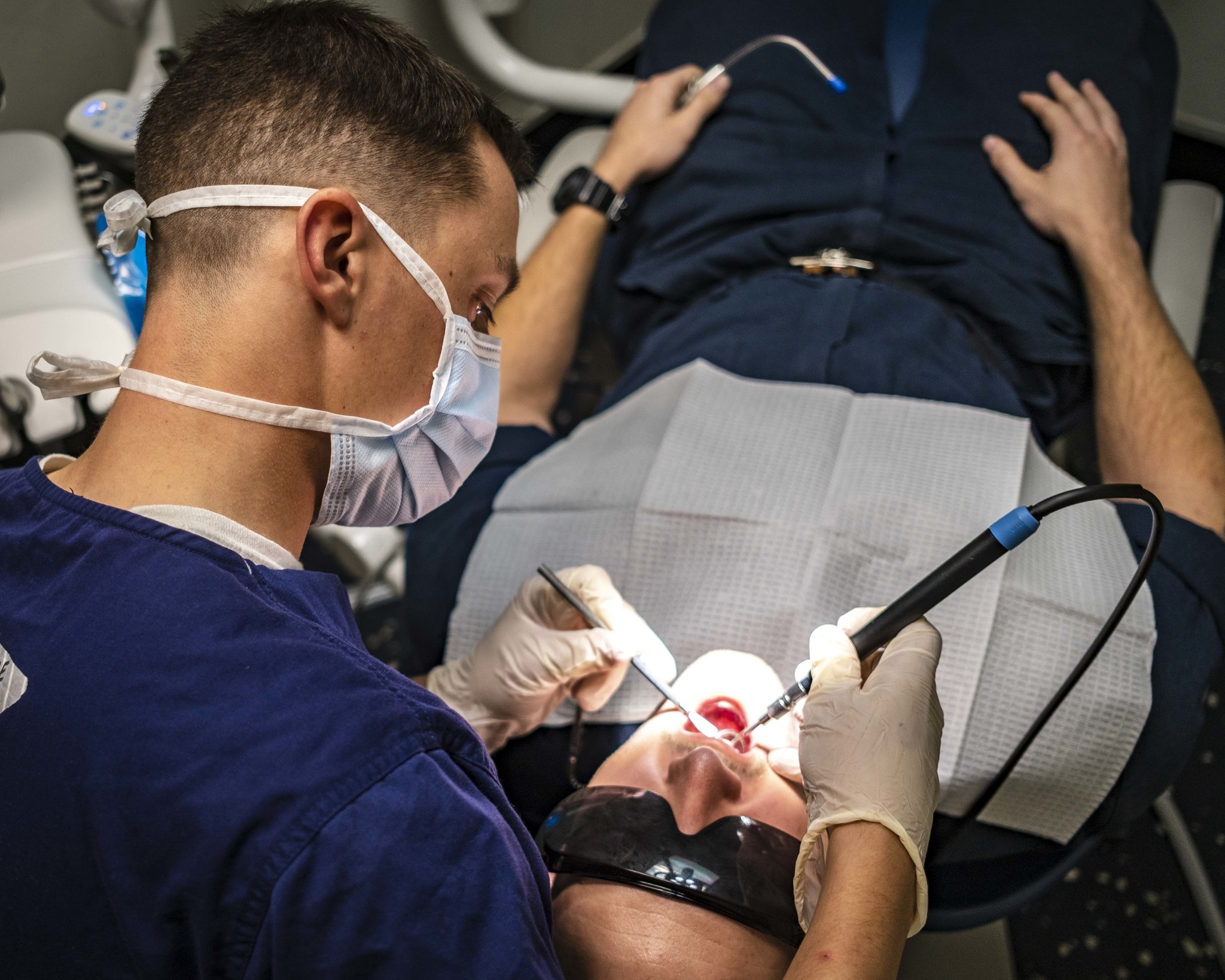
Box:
[27,183,505,526]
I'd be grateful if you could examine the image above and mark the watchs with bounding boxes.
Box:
[550,164,625,221]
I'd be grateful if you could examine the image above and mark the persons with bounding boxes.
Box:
[399,1,1223,980]
[0,5,945,979]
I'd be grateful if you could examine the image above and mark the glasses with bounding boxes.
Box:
[540,786,806,944]
